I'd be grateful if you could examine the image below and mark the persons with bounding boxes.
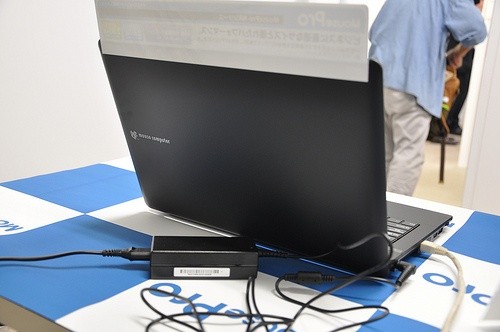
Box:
[369,0,487,197]
[427,0,483,144]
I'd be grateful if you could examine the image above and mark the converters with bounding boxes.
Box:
[148,236,259,280]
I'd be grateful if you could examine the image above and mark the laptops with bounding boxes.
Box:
[97,39,453,276]
